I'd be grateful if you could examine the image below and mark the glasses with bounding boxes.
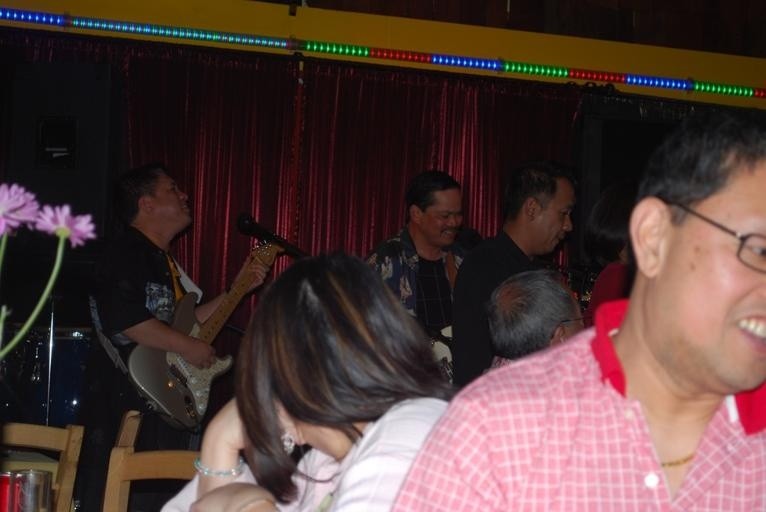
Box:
[669,199,765,275]
[550,310,592,339]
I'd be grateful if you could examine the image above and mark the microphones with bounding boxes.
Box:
[235,212,306,260]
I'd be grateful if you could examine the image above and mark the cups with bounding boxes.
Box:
[7,469,53,511]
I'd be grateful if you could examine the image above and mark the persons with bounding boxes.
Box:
[365,170,465,387]
[388,109,766,512]
[91,157,273,511]
[158,394,345,511]
[583,180,638,330]
[191,251,451,512]
[451,164,579,400]
[479,267,587,378]
[447,227,482,272]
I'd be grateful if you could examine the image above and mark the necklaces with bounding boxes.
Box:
[661,454,695,468]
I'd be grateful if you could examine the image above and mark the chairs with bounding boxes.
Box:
[101,409,204,512]
[0,421,85,512]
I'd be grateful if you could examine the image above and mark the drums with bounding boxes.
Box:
[3,324,92,415]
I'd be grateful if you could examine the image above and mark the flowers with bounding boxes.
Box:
[0,182,98,362]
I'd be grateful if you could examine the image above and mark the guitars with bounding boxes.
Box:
[129,238,286,428]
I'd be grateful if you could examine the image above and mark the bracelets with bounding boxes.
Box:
[193,455,244,477]
